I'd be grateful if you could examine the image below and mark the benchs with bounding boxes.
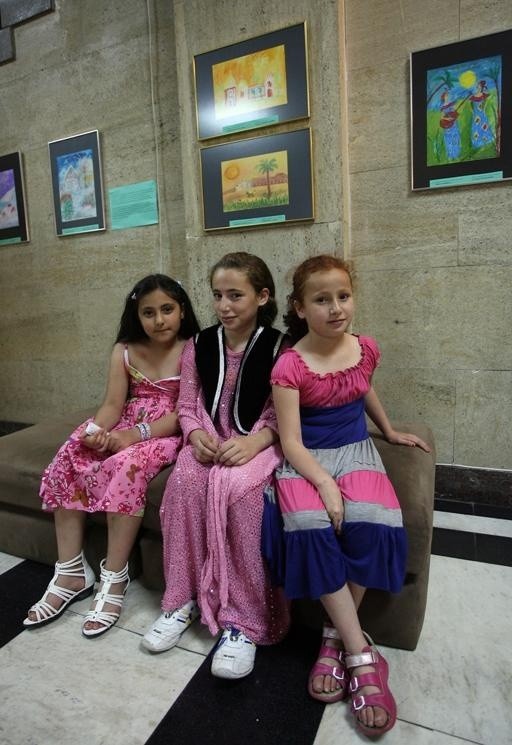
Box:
[0,405,436,652]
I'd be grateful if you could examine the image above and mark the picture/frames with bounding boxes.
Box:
[192,20,311,142]
[0,0,56,30]
[0,26,16,66]
[47,129,106,238]
[198,126,315,232]
[409,28,511,192]
[0,151,29,247]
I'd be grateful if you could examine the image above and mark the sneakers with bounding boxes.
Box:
[142,598,200,653]
[210,625,257,679]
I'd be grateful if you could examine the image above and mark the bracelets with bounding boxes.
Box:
[135,421,151,441]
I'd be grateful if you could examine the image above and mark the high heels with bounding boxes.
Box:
[344,630,397,738]
[22,550,96,629]
[307,625,350,703]
[81,557,131,638]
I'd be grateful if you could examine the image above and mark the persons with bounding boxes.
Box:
[23,275,203,637]
[271,257,429,740]
[142,253,295,681]
